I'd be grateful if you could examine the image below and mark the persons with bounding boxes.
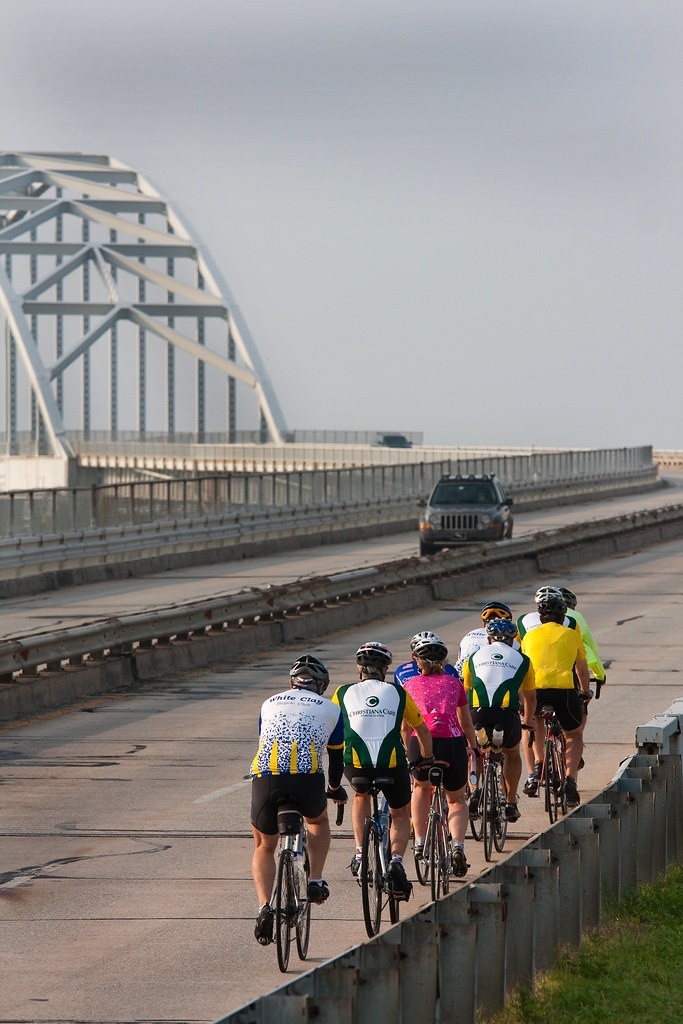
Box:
[516,587,605,809]
[394,631,483,878]
[331,642,435,902]
[252,654,345,947]
[475,486,493,504]
[455,601,537,821]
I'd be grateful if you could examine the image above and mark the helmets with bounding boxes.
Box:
[481,601,513,622]
[290,654,330,691]
[355,642,392,665]
[535,586,563,603]
[558,588,578,605]
[537,595,567,614]
[486,617,518,640]
[410,631,439,649]
[415,638,449,655]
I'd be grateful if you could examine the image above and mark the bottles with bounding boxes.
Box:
[293,852,299,885]
[378,814,389,846]
[470,771,477,784]
[494,774,501,789]
[492,725,504,753]
[475,723,491,753]
[297,852,303,886]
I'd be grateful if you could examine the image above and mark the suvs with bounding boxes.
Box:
[369,434,411,449]
[416,473,514,556]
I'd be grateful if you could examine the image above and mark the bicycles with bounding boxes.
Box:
[353,755,431,940]
[269,785,344,972]
[412,662,604,895]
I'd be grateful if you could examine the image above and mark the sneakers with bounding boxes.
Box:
[453,846,468,878]
[504,802,521,818]
[388,861,412,903]
[307,880,329,902]
[350,854,359,876]
[412,845,423,859]
[468,790,480,816]
[254,902,274,947]
[565,778,580,806]
[523,779,538,795]
[535,762,544,778]
